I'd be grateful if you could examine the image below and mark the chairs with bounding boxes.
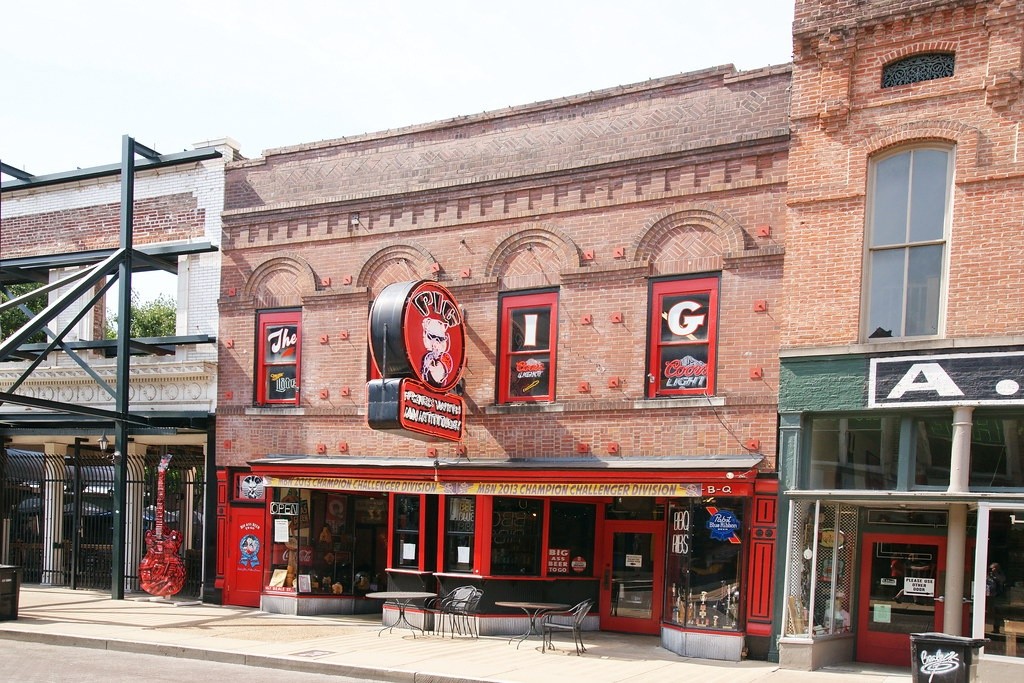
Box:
[423,584,484,641]
[541,597,596,656]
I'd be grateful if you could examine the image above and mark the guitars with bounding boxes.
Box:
[137,454,188,596]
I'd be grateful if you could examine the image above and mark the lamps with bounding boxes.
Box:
[96,430,121,463]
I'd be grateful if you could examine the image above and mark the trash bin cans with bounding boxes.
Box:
[0,563,21,622]
[909,630,991,683]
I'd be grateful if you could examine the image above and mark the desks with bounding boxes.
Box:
[364,592,438,638]
[493,601,573,652]
